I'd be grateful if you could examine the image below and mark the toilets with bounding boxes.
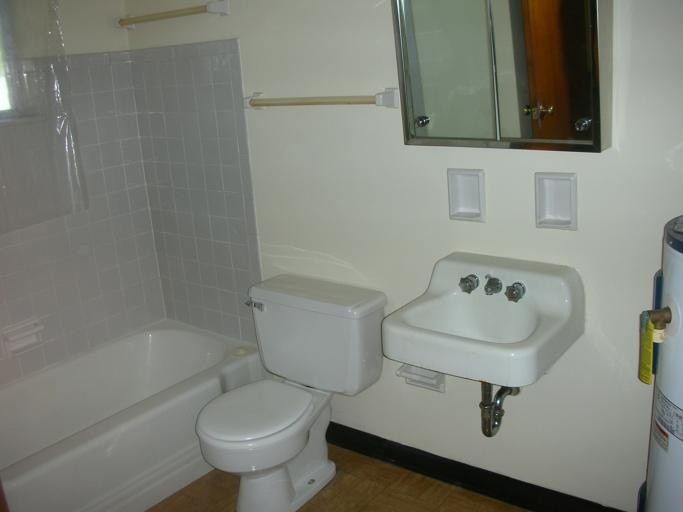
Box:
[194,272,388,512]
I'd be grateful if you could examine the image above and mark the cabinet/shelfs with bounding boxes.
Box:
[116,1,400,109]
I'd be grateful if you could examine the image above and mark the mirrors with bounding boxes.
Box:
[391,0,612,153]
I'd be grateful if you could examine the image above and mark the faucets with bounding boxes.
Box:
[380,251,586,389]
[483,278,503,297]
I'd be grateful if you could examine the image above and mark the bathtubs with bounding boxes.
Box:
[0,317,284,512]
